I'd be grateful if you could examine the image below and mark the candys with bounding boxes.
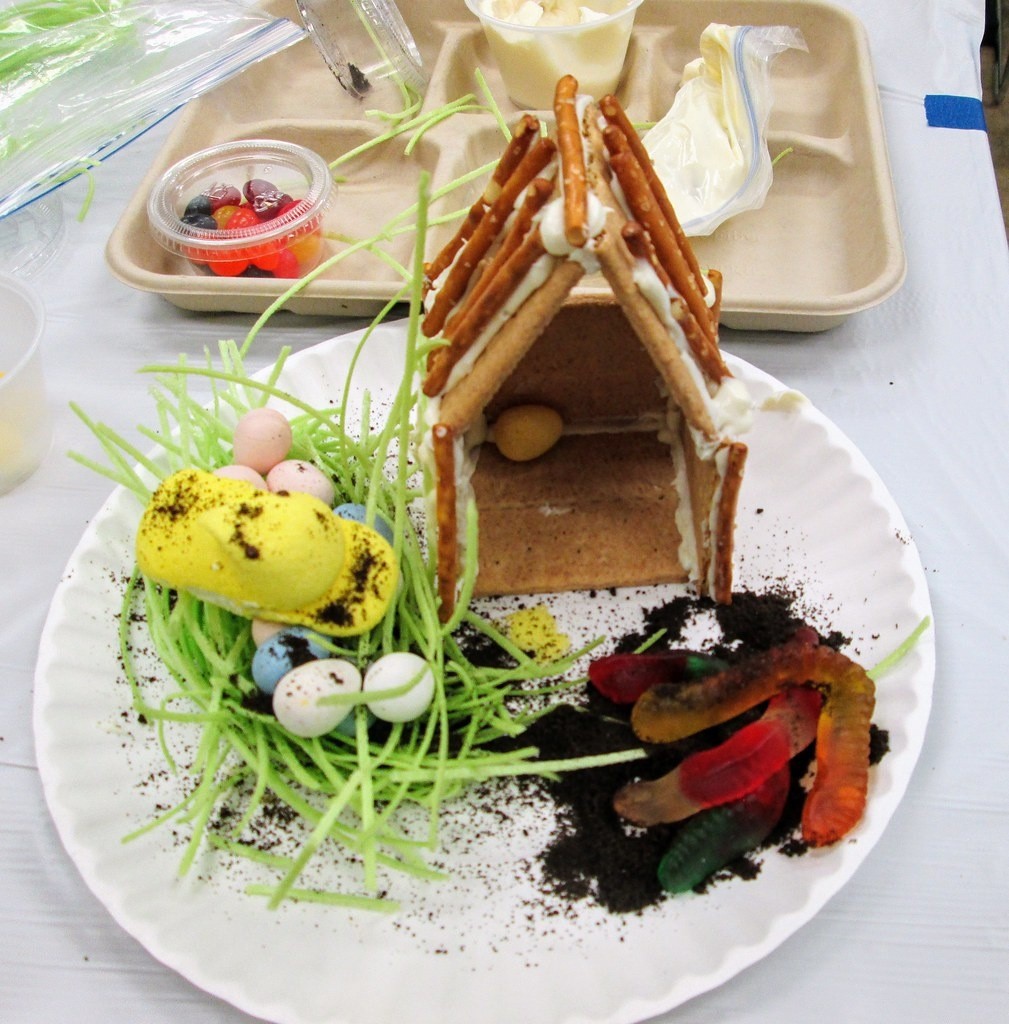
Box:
[179,178,322,282]
[590,625,876,893]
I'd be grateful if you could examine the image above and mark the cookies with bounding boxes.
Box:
[413,75,746,620]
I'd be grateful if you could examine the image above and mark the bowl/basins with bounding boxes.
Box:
[145,139,338,278]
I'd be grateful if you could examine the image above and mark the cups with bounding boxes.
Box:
[462,0,646,112]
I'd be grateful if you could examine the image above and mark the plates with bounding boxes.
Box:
[31,315,936,1024]
